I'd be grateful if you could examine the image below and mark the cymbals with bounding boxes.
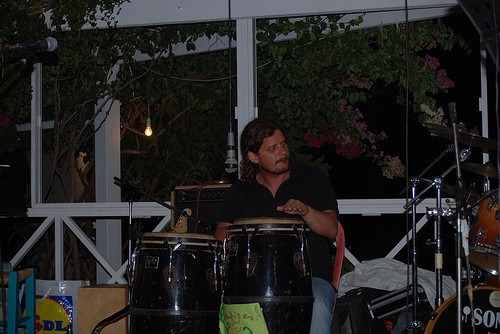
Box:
[422,121,500,151]
[451,160,500,179]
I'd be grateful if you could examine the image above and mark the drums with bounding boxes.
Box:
[464,189,482,205]
[132,226,222,334]
[220,217,316,334]
[466,191,500,275]
[424,276,500,334]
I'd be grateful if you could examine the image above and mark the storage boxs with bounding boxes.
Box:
[77,284,128,334]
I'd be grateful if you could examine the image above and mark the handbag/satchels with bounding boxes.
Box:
[18,279,91,334]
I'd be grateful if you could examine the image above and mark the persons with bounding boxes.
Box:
[208,118,339,334]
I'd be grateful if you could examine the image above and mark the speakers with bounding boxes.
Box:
[173,189,231,232]
